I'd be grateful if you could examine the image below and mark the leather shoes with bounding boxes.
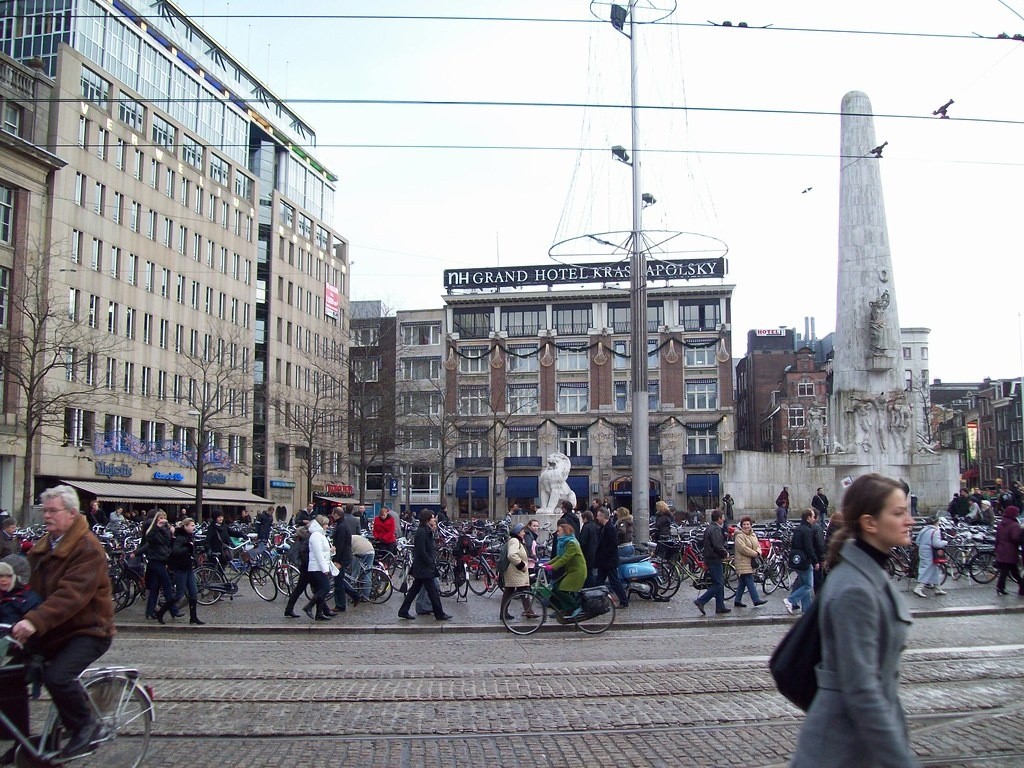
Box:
[0,745,20,766]
[62,719,101,757]
[913,587,948,597]
[398,611,453,620]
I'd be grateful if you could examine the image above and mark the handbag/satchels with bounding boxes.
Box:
[788,549,809,570]
[768,596,821,713]
[931,529,948,563]
[286,540,310,566]
[222,543,234,561]
[750,557,763,569]
[330,561,340,576]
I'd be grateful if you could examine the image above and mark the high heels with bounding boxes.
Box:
[996,588,1009,596]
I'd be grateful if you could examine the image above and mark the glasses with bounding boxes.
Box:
[38,508,68,516]
[39,507,70,516]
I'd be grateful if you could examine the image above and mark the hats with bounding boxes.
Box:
[981,500,991,506]
[21,542,33,551]
[1004,505,1019,518]
[510,523,525,534]
[211,510,224,520]
[0,553,31,593]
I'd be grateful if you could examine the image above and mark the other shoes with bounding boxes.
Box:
[692,598,803,615]
[500,611,540,619]
[563,607,582,618]
[614,604,625,609]
[282,594,362,620]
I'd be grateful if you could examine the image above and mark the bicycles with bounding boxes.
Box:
[0,620,156,768]
[0,510,1024,637]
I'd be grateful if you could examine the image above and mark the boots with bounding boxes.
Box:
[145,597,205,625]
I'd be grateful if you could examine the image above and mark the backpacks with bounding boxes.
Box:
[498,537,521,571]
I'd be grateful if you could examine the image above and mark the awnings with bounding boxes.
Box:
[317,497,360,504]
[455,477,489,498]
[60,480,275,507]
[565,476,589,499]
[505,477,538,497]
[687,475,719,496]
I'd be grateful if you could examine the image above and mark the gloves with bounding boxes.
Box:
[543,562,552,571]
[206,548,212,556]
[241,533,249,540]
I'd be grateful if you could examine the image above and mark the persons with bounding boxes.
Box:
[868,290,891,355]
[781,506,852,615]
[734,517,768,608]
[806,400,825,453]
[0,486,275,767]
[776,487,789,531]
[721,494,734,522]
[654,500,676,543]
[285,500,453,619]
[811,487,828,531]
[911,479,1024,599]
[791,473,919,768]
[694,510,731,616]
[500,498,635,618]
[848,394,914,454]
